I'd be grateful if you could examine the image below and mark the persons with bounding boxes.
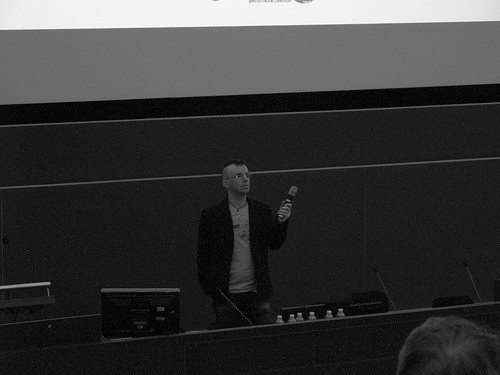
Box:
[197,159,293,320]
[397,316,500,375]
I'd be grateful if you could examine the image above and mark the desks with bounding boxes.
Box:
[0,303,500,375]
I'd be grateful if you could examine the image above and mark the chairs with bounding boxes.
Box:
[207,291,473,330]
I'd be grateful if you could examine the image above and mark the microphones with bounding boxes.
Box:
[215,287,254,326]
[278,185,298,220]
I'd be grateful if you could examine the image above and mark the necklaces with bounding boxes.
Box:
[229,200,247,211]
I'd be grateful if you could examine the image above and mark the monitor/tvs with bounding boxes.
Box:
[100,288,181,341]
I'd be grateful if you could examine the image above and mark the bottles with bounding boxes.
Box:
[276,315,284,324]
[336,309,345,317]
[295,313,304,321]
[287,314,296,322]
[308,312,316,320]
[325,310,333,319]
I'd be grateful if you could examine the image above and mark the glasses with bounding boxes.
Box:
[226,173,253,180]
